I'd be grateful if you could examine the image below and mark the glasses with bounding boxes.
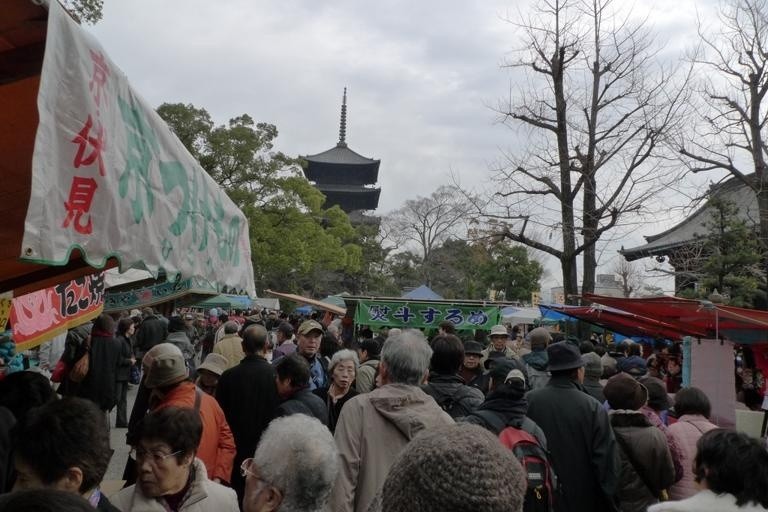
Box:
[241,458,268,482]
[135,450,183,464]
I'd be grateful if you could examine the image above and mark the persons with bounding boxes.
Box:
[2,304,768,510]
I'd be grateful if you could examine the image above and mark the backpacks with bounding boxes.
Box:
[473,410,564,512]
[426,382,472,418]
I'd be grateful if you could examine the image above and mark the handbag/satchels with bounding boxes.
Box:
[128,366,141,385]
[68,351,89,384]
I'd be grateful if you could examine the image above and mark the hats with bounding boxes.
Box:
[367,421,528,512]
[358,338,381,353]
[465,325,675,411]
[198,353,228,377]
[297,319,326,338]
[142,343,186,388]
